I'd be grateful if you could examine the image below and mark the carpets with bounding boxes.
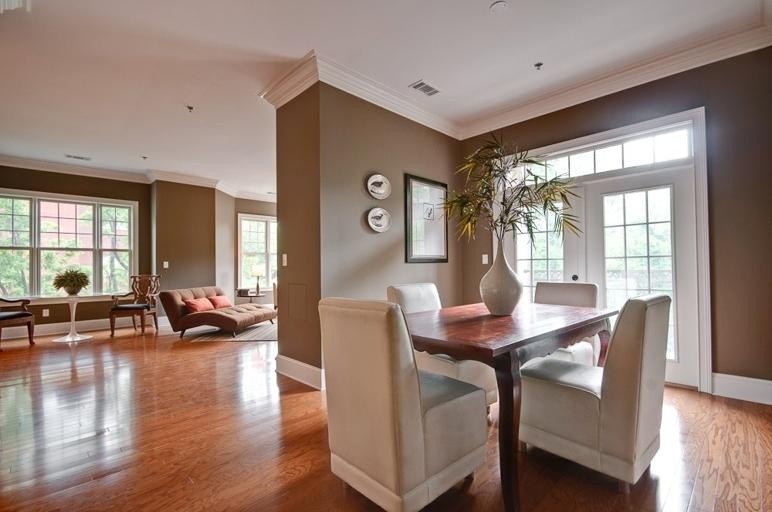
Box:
[190,319,278,343]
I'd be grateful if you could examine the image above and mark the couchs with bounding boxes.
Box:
[159,286,276,337]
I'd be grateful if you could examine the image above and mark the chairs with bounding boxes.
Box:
[318,297,487,512]
[0,298,35,351]
[513,282,602,381]
[387,283,498,425]
[109,275,158,337]
[518,294,671,495]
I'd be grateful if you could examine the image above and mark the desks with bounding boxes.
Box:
[40,294,108,342]
[404,302,619,512]
[237,294,265,303]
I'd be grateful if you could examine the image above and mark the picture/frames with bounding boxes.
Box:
[404,172,448,264]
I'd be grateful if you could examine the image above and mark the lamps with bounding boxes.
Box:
[250,264,265,295]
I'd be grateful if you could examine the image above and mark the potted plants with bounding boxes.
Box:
[438,129,588,316]
[51,269,91,295]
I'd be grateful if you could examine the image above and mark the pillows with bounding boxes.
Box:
[208,296,232,308]
[183,298,214,313]
